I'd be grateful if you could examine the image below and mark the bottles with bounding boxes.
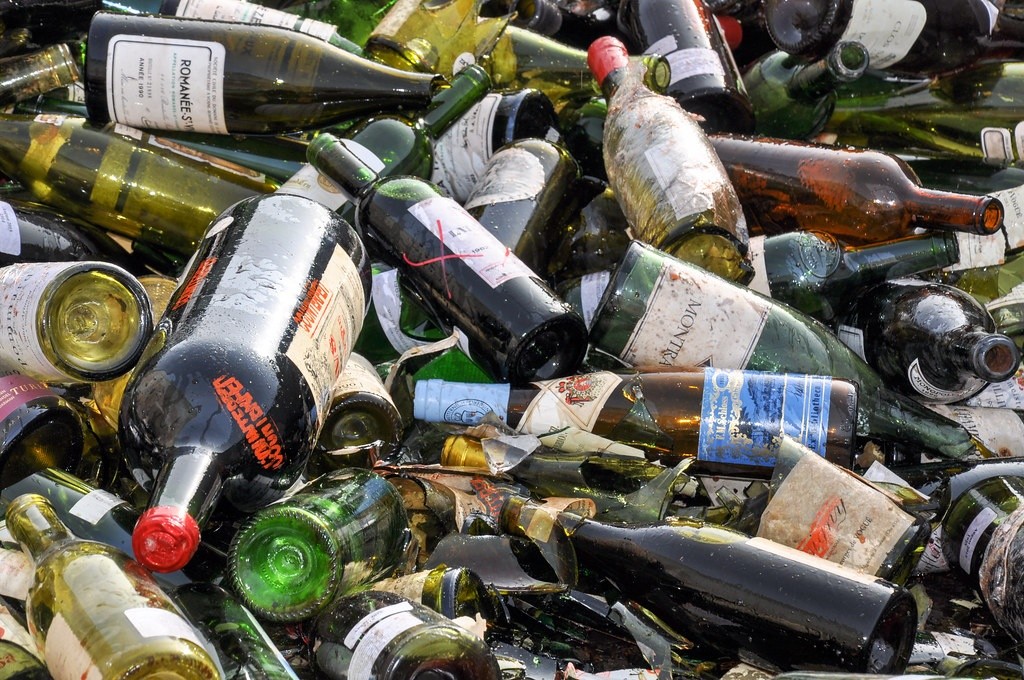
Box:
[0,0,1024,680]
